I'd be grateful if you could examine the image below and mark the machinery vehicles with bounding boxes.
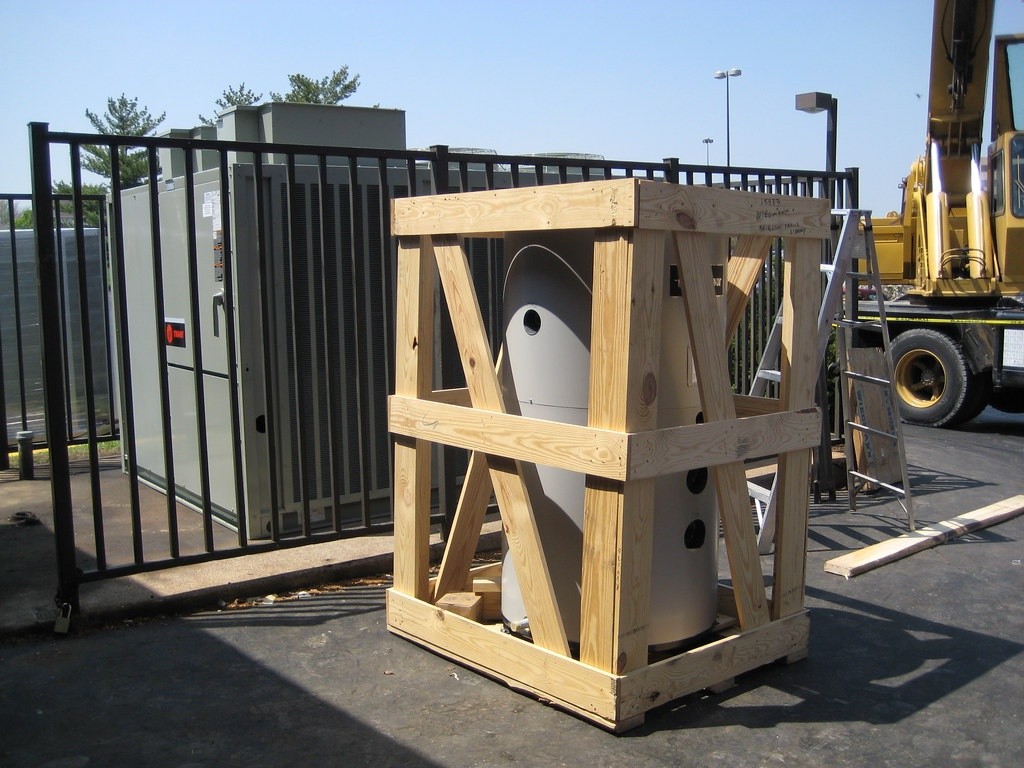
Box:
[831,2,1023,437]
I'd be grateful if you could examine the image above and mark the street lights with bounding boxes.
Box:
[795,92,838,212]
[702,138,714,167]
[714,69,742,168]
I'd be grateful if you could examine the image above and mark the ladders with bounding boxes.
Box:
[716,204,915,559]
